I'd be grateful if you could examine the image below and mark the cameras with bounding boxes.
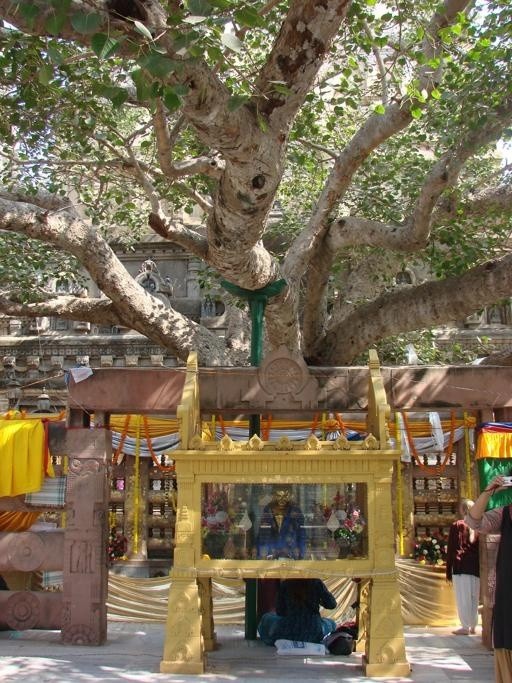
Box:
[500,476,512,486]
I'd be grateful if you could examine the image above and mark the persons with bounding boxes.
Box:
[257,576,337,646]
[257,484,306,559]
[446,500,481,636]
[465,469,512,683]
